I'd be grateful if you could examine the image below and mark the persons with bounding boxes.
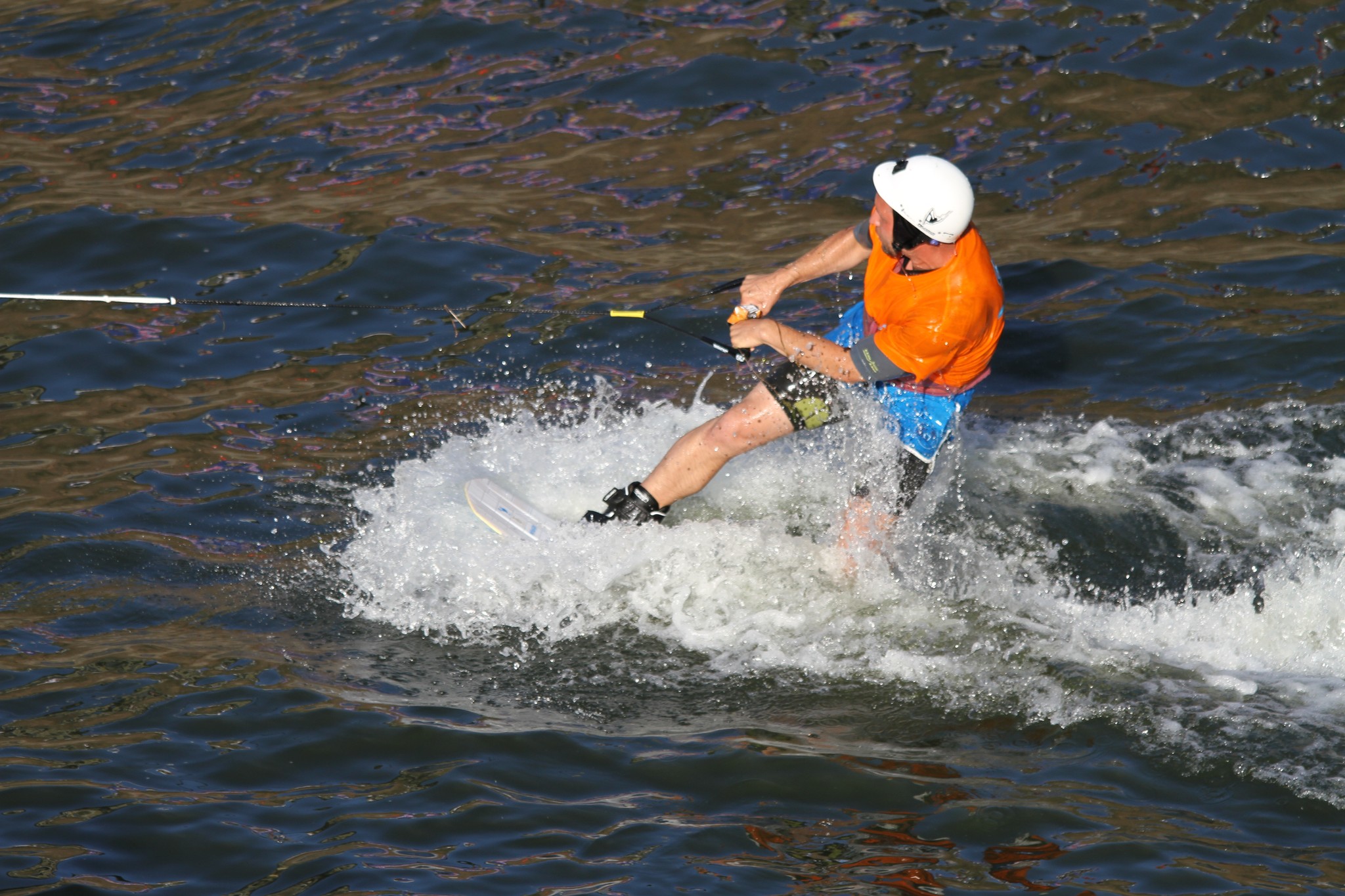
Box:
[586,155,1004,572]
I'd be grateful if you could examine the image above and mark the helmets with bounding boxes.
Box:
[873,154,974,243]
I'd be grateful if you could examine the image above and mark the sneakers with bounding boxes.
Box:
[580,481,669,529]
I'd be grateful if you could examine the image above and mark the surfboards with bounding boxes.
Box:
[465,477,569,544]
